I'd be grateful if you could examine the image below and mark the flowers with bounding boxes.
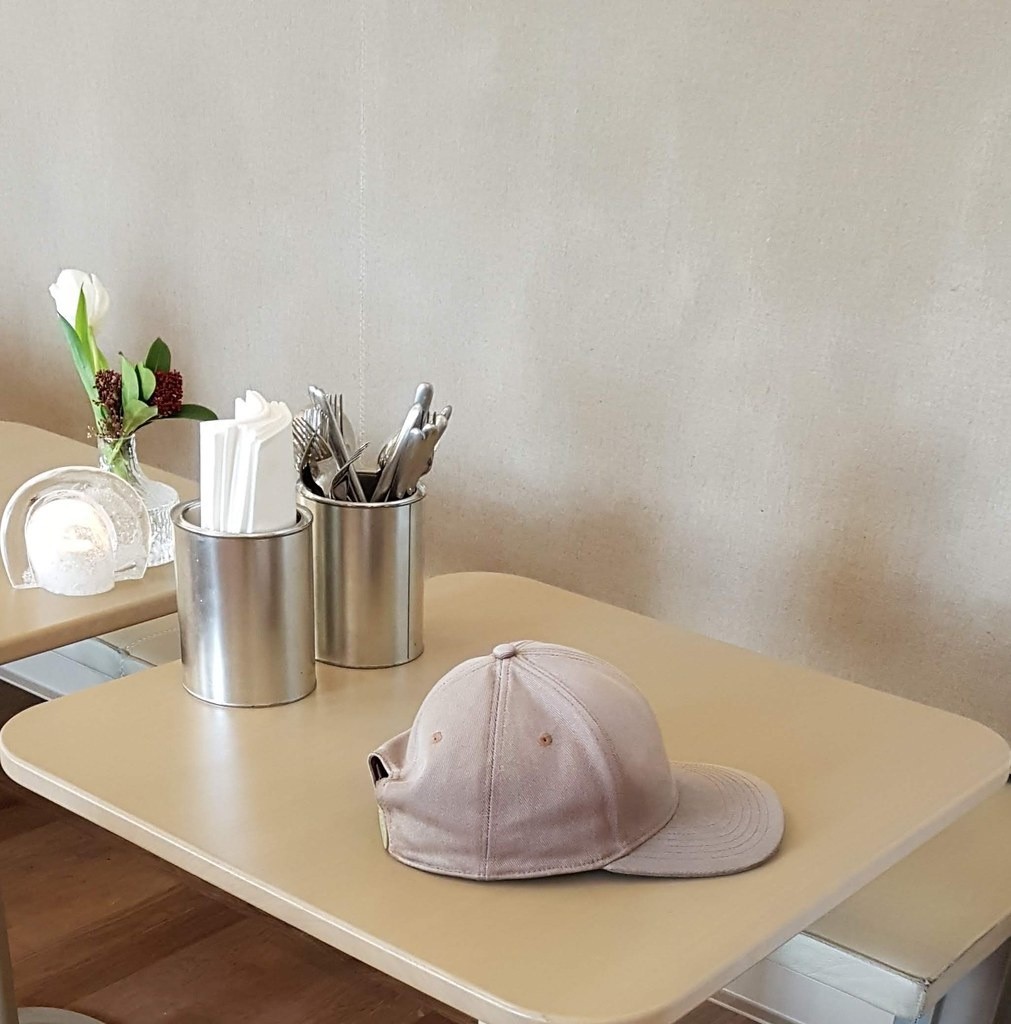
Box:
[48,268,218,484]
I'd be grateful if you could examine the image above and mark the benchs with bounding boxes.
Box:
[0,609,1011,1024]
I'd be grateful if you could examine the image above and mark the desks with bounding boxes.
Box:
[0,571,1011,1024]
[0,419,200,666]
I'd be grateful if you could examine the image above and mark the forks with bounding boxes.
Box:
[407,413,436,497]
[292,385,369,503]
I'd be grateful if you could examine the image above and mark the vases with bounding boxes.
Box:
[97,434,181,568]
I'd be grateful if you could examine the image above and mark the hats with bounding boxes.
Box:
[367,640,786,882]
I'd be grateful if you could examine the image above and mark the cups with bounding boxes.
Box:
[296,472,429,668]
[170,498,316,706]
